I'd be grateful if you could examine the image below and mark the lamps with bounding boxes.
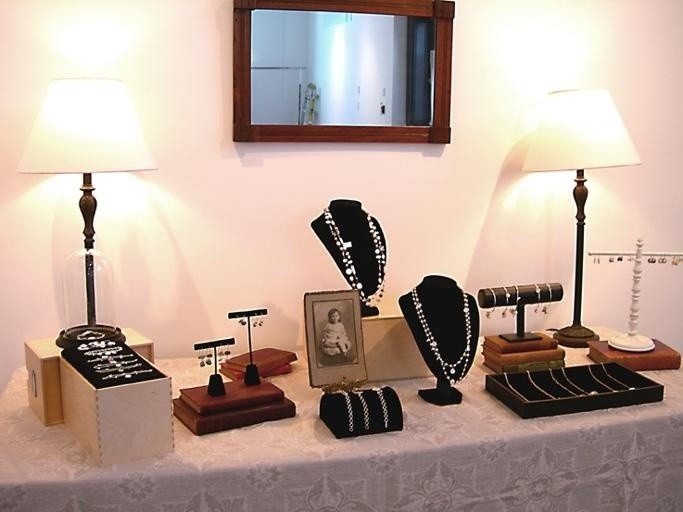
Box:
[18,78,159,349]
[522,86,640,348]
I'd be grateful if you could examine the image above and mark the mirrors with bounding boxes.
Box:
[232,1,455,144]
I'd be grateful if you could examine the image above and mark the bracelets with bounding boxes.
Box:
[484,282,554,319]
[74,330,154,381]
[334,387,392,432]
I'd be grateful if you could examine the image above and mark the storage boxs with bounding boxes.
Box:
[24,328,156,427]
[58,344,176,468]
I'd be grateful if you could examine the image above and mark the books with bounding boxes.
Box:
[219,363,292,382]
[171,397,296,436]
[483,330,559,353]
[480,343,565,365]
[178,375,284,414]
[585,339,681,373]
[225,345,297,375]
[479,352,565,375]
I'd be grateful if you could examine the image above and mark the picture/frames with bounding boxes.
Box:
[303,289,368,389]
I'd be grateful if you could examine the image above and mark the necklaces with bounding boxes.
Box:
[409,286,473,374]
[503,361,636,401]
[320,206,381,302]
[409,286,472,388]
[321,206,385,309]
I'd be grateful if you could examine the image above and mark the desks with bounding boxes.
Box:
[0,326,682,512]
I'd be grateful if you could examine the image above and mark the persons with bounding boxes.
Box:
[319,308,352,358]
